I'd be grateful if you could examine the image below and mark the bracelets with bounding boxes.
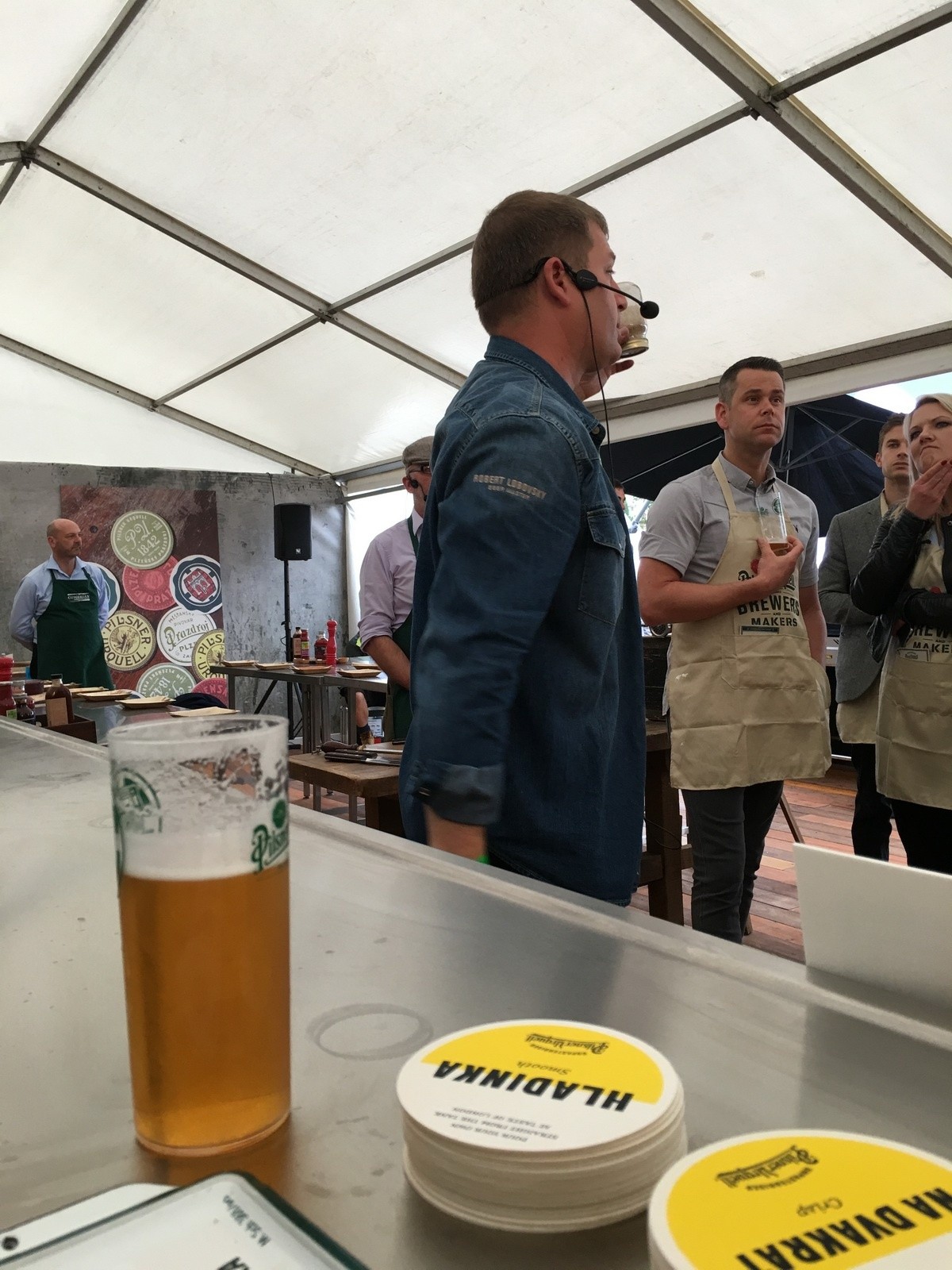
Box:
[476,856,490,865]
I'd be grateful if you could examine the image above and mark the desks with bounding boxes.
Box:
[0,713,952,1270]
[284,738,408,830]
[211,650,389,823]
[636,717,696,926]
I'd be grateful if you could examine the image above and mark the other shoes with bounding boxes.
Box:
[359,729,375,745]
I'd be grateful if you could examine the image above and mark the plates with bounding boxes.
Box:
[353,663,379,669]
[76,690,133,701]
[114,696,177,709]
[69,687,109,698]
[336,668,382,678]
[165,706,240,718]
[291,666,334,674]
[44,683,83,691]
[220,660,259,666]
[254,662,294,670]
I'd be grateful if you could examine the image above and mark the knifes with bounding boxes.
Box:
[320,741,403,767]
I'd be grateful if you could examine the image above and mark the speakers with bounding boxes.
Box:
[272,502,312,562]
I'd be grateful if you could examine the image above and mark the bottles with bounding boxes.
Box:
[0,680,35,721]
[300,628,310,660]
[45,673,74,726]
[292,626,302,661]
[617,281,649,358]
[1,653,6,657]
[314,630,329,661]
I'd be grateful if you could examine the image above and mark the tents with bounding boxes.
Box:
[599,384,923,539]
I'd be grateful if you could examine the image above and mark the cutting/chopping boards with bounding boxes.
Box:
[0,679,44,695]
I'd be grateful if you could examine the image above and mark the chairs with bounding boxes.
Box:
[783,825,952,1003]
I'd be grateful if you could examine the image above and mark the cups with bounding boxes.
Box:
[755,491,789,553]
[106,713,291,1157]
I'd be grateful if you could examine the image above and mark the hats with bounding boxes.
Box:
[402,436,435,470]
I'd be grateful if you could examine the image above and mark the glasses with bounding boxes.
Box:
[408,463,431,474]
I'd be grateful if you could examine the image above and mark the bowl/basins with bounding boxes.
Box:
[338,657,349,663]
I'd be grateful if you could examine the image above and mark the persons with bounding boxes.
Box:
[399,191,644,906]
[9,517,124,748]
[357,357,952,945]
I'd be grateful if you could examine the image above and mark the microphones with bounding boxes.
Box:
[575,270,660,320]
[411,478,428,501]
